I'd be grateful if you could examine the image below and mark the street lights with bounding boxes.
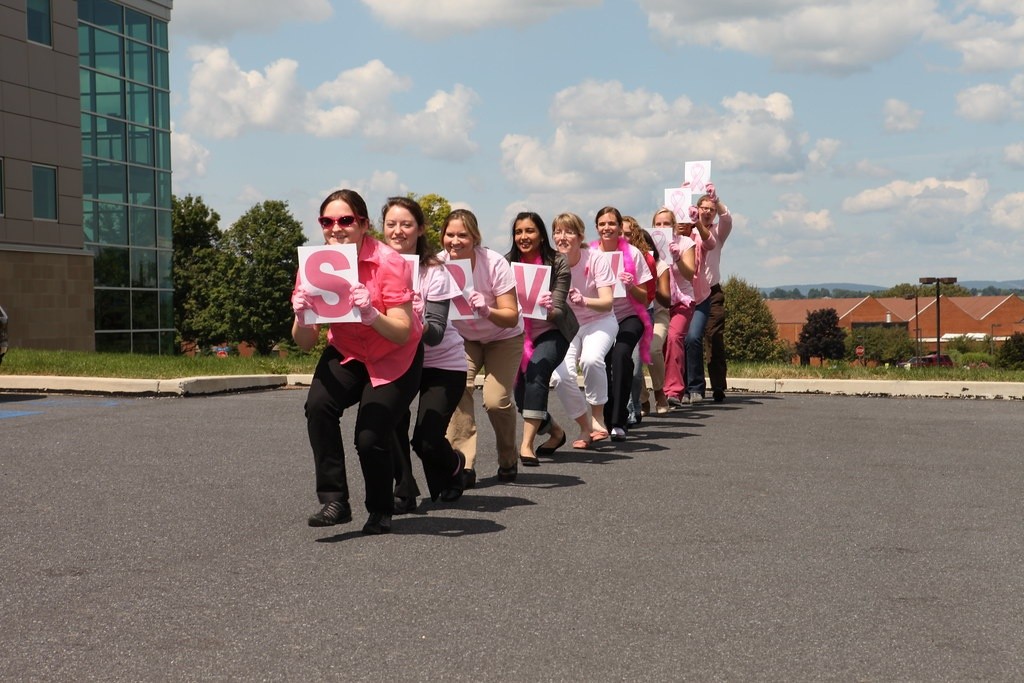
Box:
[919,277,957,367]
[903,294,921,368]
[990,323,1002,354]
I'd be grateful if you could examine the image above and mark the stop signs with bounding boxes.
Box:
[856,345,865,356]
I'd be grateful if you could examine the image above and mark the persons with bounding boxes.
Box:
[291,189,424,535]
[382,181,733,515]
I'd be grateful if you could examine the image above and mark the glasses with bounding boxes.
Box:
[319,216,366,227]
[699,207,717,213]
[553,231,578,237]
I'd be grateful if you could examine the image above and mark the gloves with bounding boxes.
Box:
[569,288,587,308]
[681,182,690,188]
[290,293,317,328]
[469,291,490,319]
[706,182,718,203]
[689,206,698,224]
[538,291,554,312]
[669,242,679,261]
[350,283,380,326]
[412,295,427,326]
[619,272,634,292]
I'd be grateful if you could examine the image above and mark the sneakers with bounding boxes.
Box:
[309,501,352,526]
[363,498,394,534]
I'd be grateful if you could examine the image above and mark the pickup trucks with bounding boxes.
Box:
[898,354,954,369]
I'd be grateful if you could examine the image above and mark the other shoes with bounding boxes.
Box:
[464,468,477,491]
[498,461,517,482]
[713,390,725,402]
[655,392,705,413]
[520,454,540,466]
[535,430,566,455]
[394,496,417,514]
[611,400,650,442]
[439,449,465,500]
[573,430,608,448]
